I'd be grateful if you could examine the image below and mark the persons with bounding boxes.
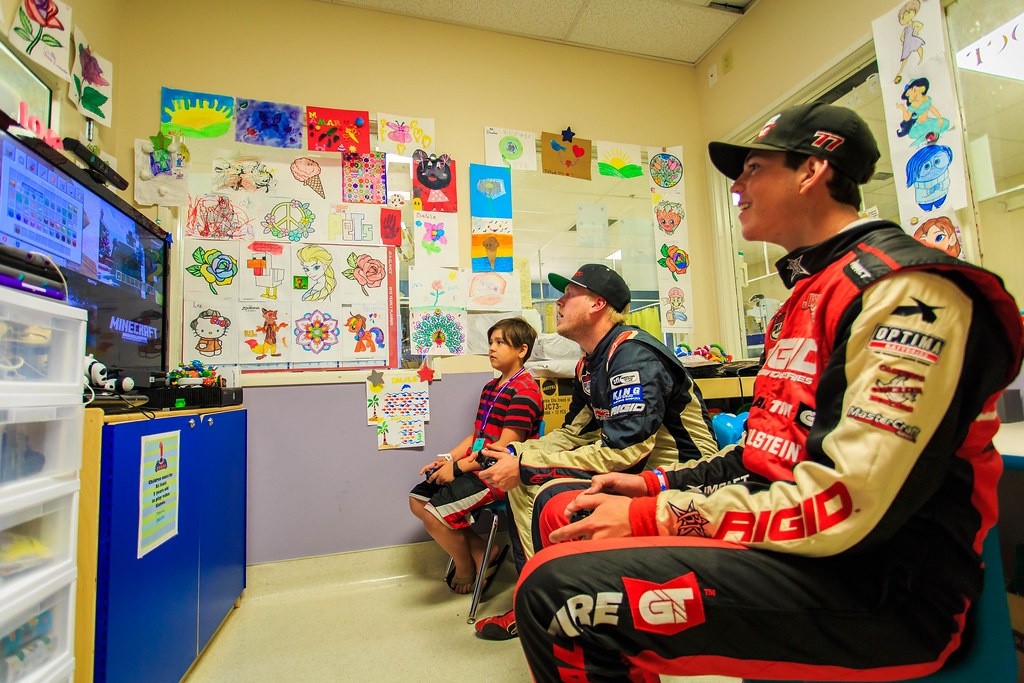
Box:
[410,318,544,602]
[515,102,1024,683]
[473,264,722,641]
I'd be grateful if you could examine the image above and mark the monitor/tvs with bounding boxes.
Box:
[0,111,171,415]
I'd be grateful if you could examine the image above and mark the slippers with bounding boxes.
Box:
[446,566,476,594]
[475,543,510,599]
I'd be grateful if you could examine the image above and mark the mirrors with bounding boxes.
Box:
[531,191,664,345]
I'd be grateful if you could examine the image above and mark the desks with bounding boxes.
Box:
[692,377,757,399]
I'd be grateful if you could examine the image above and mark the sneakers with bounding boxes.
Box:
[475,609,519,640]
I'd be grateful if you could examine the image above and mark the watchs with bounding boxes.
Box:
[453,461,463,478]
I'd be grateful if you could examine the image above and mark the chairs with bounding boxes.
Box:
[444,419,545,624]
[710,411,1017,683]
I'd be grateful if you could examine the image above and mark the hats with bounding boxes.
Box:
[548,263,631,313]
[708,102,881,181]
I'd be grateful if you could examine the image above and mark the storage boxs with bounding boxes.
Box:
[0,285,89,683]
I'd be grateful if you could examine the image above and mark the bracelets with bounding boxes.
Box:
[437,454,454,461]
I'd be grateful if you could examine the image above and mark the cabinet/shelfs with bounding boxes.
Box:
[74,403,248,683]
